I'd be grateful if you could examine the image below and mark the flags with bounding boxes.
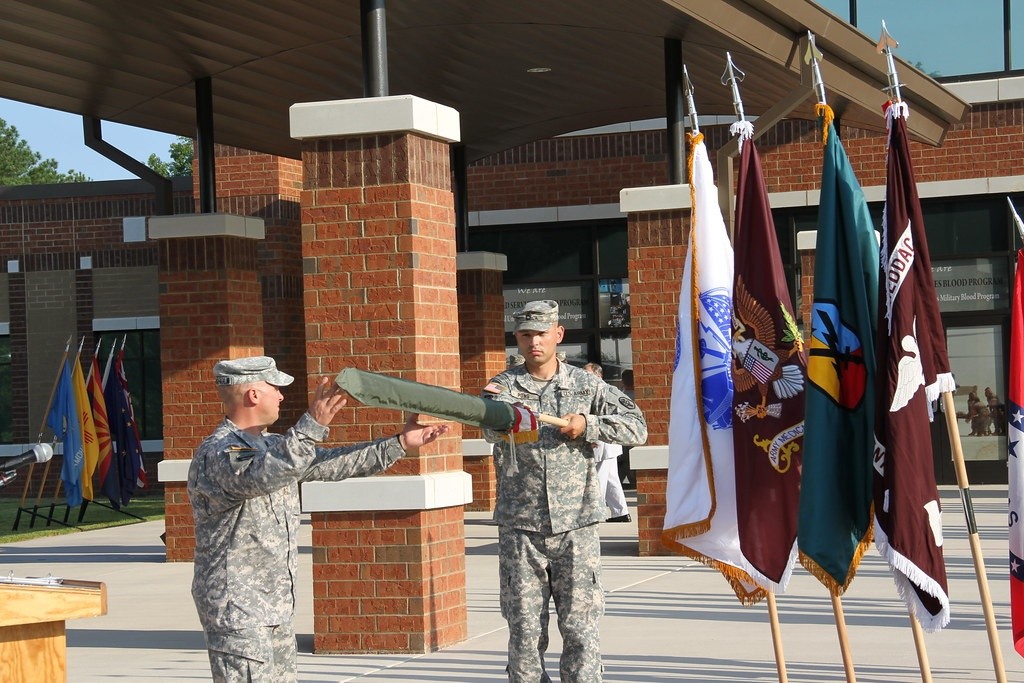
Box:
[730,120,808,595]
[661,135,768,609]
[871,100,957,636]
[797,101,879,599]
[45,348,149,512]
[1007,246,1024,658]
[334,367,538,443]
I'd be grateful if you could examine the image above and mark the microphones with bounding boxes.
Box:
[0,443,54,472]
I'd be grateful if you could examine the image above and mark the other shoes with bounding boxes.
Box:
[606,514,632,523]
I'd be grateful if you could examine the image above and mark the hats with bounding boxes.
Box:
[512,300,559,336]
[510,354,526,366]
[557,350,567,362]
[212,356,295,387]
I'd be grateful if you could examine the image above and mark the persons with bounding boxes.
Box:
[964,387,1005,437]
[556,351,568,362]
[510,354,525,370]
[480,299,649,683]
[582,363,633,523]
[617,369,636,489]
[187,354,451,683]
